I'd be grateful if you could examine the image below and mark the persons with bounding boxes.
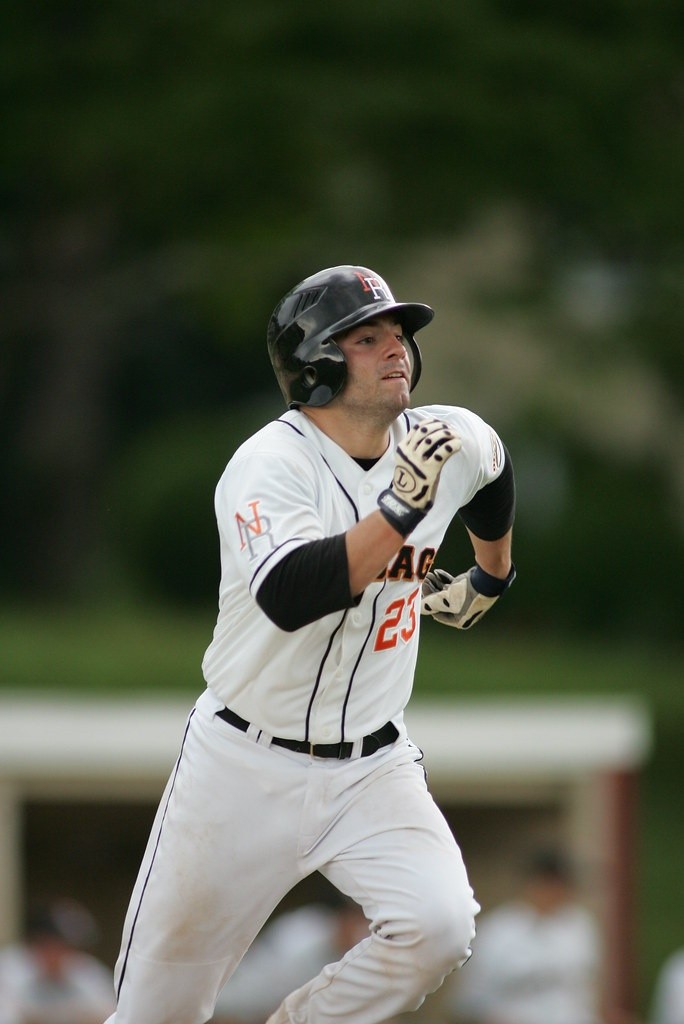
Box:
[469,852,601,1021]
[109,265,519,1023]
[0,921,104,1021]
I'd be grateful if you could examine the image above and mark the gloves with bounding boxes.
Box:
[376,418,463,537]
[420,556,517,629]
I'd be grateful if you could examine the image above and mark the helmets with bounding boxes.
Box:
[267,264,434,409]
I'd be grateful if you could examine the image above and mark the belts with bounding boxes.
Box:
[216,707,398,761]
